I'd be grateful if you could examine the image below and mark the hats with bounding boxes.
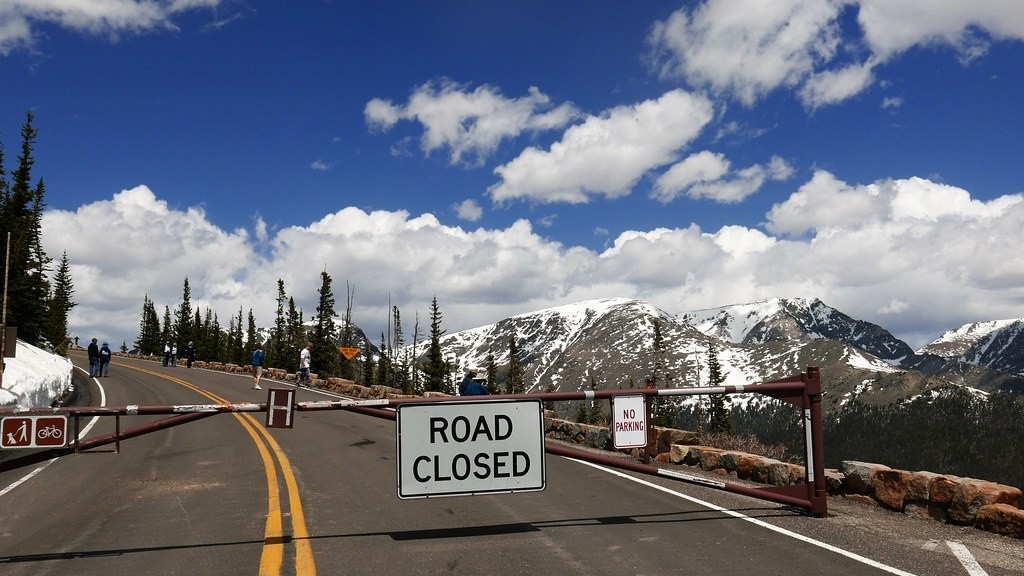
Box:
[472,373,487,380]
[469,369,477,377]
[102,342,109,348]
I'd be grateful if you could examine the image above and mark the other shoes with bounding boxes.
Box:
[90,374,99,378]
[162,364,167,367]
[104,374,110,377]
[254,385,262,390]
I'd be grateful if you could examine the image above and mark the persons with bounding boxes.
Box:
[73,335,80,345]
[459,369,489,396]
[296,343,313,386]
[162,338,196,368]
[88,338,111,378]
[250,343,264,390]
